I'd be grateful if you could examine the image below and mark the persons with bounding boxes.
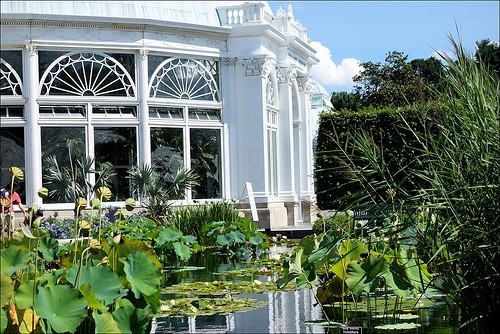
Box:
[0,184,12,201]
[0,180,30,232]
[24,207,45,226]
